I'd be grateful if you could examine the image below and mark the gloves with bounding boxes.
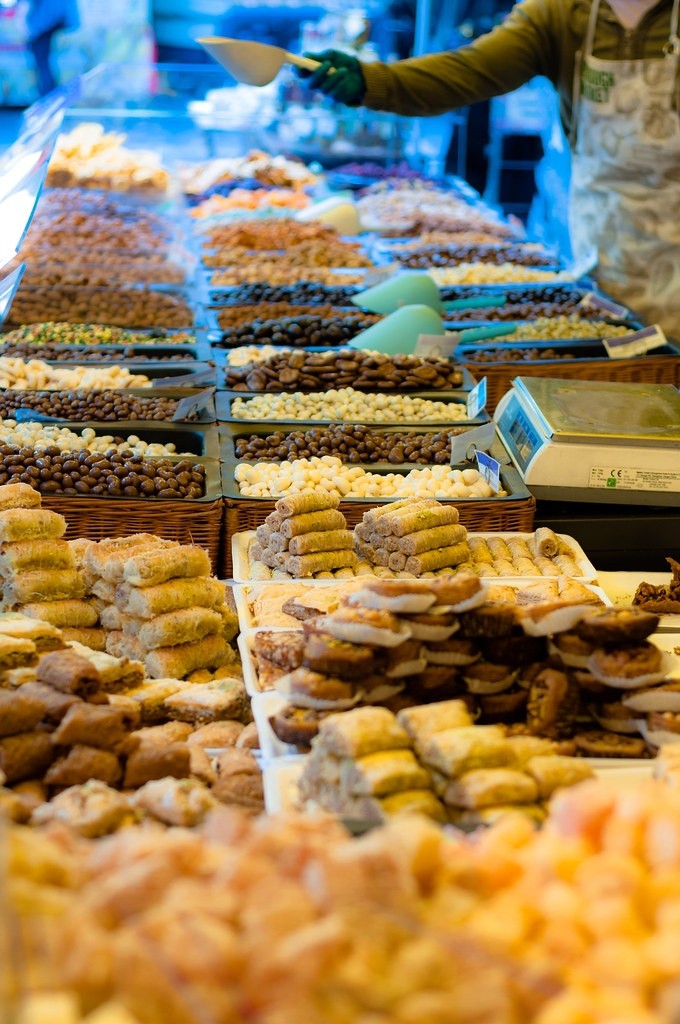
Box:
[292,50,367,108]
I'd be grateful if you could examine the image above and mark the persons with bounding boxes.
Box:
[292,0,680,347]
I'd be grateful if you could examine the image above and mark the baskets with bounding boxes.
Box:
[456,337,680,422]
[224,467,535,581]
[36,456,223,580]
